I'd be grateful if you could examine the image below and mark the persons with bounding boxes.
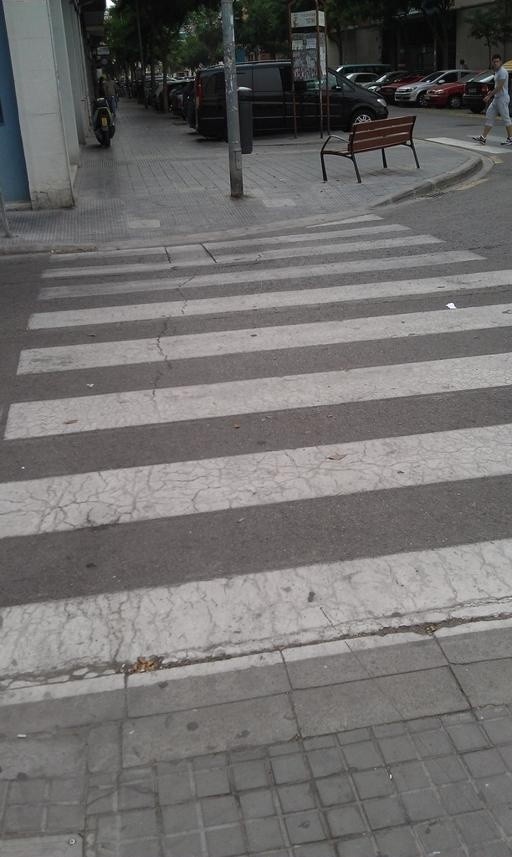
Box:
[460,58,471,78]
[98,73,119,117]
[472,54,512,146]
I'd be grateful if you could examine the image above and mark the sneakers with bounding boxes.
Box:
[500,136,512,146]
[472,134,487,145]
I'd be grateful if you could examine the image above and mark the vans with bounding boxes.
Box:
[191,54,391,139]
[336,63,407,75]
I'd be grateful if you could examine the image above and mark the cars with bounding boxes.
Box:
[342,70,512,112]
[117,68,194,114]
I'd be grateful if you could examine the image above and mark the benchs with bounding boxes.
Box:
[319,114,421,186]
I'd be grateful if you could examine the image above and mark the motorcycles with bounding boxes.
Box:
[90,94,119,151]
